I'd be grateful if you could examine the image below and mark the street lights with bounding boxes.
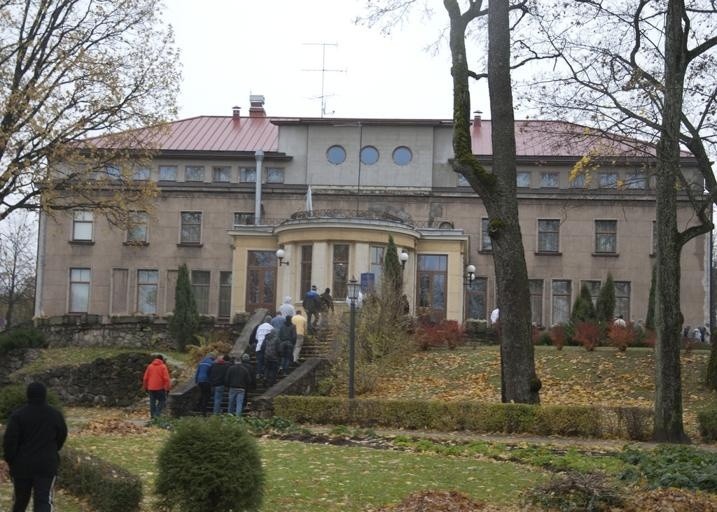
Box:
[345,274,364,399]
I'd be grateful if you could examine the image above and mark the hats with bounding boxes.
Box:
[27,382,46,400]
[208,352,249,364]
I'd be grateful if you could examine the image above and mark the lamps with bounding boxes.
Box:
[398,250,408,269]
[274,243,290,267]
[464,264,477,289]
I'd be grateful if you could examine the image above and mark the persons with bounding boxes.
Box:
[491,306,499,325]
[195,296,308,418]
[302,285,324,331]
[682,321,711,343]
[319,288,337,327]
[0,382,68,512]
[614,313,645,333]
[142,354,171,420]
